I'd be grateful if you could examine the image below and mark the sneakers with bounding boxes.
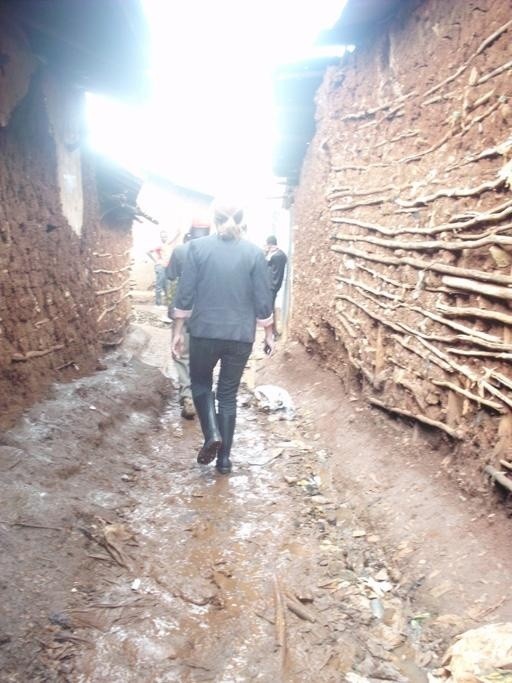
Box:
[181,402,196,418]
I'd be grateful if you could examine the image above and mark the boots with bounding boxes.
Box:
[216,413,235,474]
[194,391,223,463]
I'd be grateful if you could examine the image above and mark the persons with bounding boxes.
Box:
[168,204,275,474]
[261,235,287,342]
[146,229,181,305]
